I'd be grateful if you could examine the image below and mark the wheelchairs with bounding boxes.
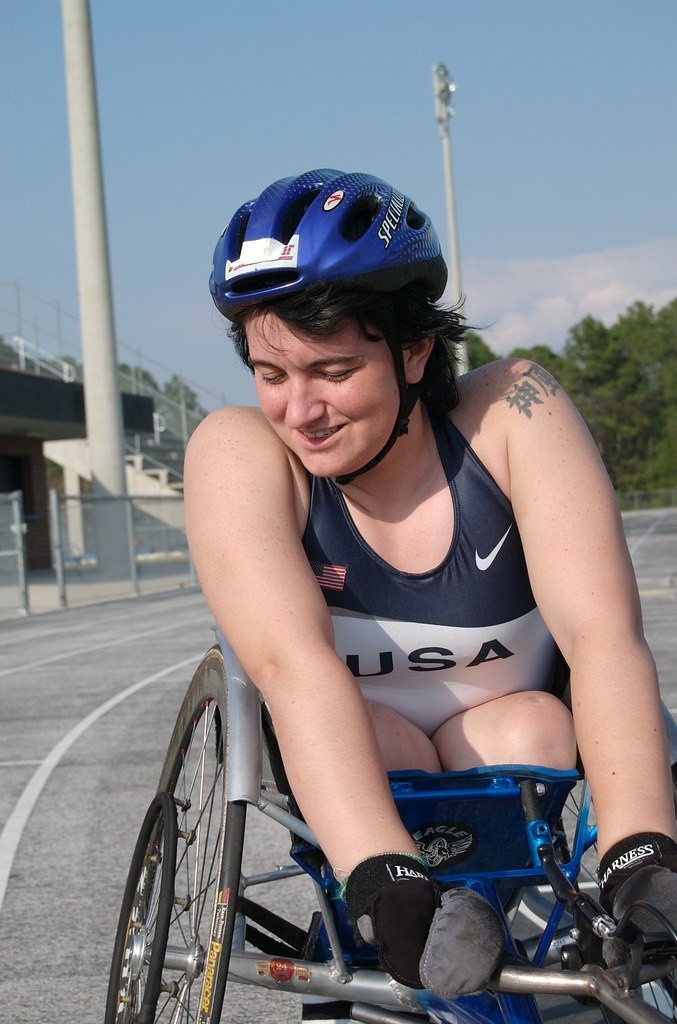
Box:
[103,625,677,1024]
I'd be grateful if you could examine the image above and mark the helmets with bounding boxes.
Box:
[208,168,448,324]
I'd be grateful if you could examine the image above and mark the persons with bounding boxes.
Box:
[184,168,677,999]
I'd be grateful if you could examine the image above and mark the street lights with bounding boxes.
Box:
[432,62,470,377]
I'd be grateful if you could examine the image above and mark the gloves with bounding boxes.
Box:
[344,853,506,995]
[596,831,677,969]
